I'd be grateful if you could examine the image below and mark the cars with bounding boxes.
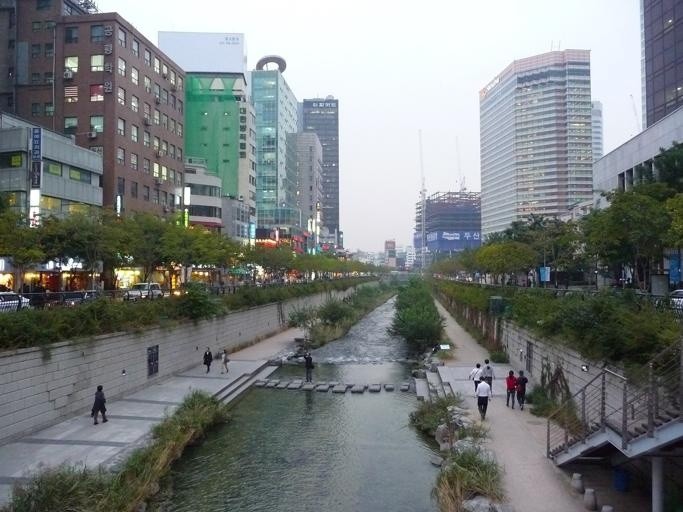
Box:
[654,289,683,311]
[44,291,99,310]
[1,290,32,313]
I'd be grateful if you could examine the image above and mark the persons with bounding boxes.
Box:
[303,353,312,382]
[203,347,213,374]
[475,376,491,421]
[18,279,45,310]
[220,349,228,374]
[505,370,516,409]
[468,363,482,398]
[516,370,529,411]
[93,385,108,425]
[482,359,496,396]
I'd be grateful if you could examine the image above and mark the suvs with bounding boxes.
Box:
[123,282,165,302]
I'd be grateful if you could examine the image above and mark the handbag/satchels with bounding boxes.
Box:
[515,384,523,393]
